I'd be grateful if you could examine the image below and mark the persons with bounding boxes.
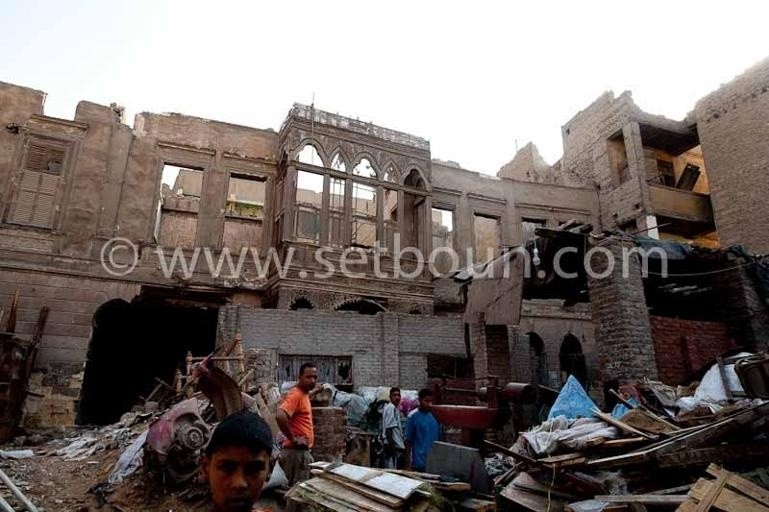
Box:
[379,386,406,468]
[275,359,318,491]
[205,405,278,512]
[610,385,639,419]
[402,388,441,473]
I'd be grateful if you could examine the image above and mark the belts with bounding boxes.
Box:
[280,445,310,451]
